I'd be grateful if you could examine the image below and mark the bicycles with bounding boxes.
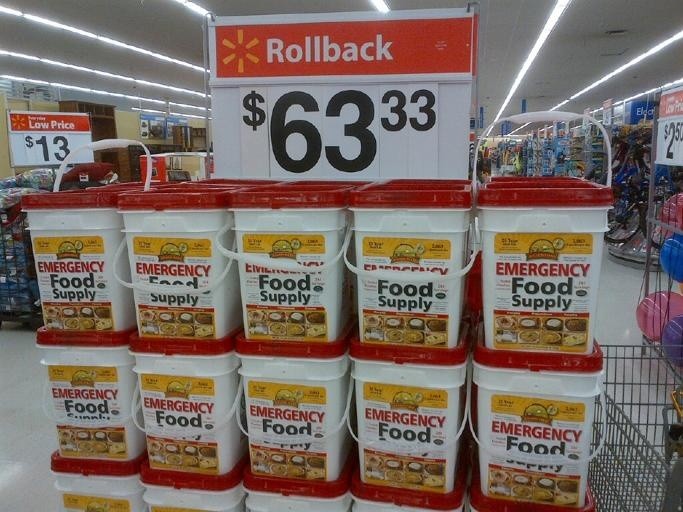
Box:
[585,126,683,248]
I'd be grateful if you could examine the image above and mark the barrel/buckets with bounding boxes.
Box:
[350,342,470,495]
[232,339,349,482]
[48,470,448,512]
[228,179,367,345]
[464,344,612,509]
[353,178,477,349]
[126,336,242,483]
[31,327,142,476]
[16,183,136,334]
[478,173,608,354]
[119,176,279,349]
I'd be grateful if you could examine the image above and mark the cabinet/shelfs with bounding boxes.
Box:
[531,104,682,391]
